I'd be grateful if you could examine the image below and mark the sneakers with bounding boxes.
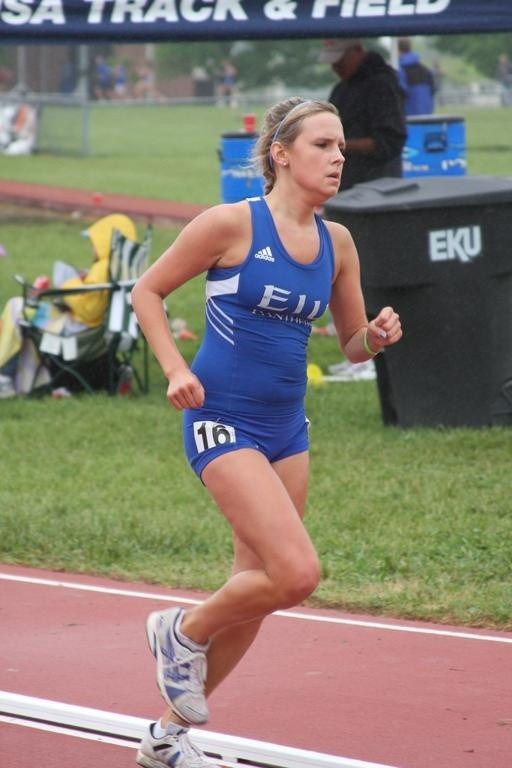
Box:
[1,380,16,399]
[144,607,209,726]
[135,721,221,768]
[328,358,374,376]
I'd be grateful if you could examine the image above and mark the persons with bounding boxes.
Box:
[0,213,138,398]
[496,53,512,107]
[394,38,435,116]
[315,37,408,193]
[128,97,406,767]
[91,54,240,109]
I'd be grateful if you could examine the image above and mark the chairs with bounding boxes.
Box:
[15,225,152,397]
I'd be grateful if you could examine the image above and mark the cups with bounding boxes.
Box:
[244,115,256,135]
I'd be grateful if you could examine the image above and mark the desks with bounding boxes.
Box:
[220,115,467,205]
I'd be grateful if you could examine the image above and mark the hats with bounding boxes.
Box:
[318,38,358,65]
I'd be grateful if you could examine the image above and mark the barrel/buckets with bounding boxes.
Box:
[399,114,467,177]
[217,133,265,201]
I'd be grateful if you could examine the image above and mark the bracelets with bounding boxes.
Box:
[363,330,383,356]
